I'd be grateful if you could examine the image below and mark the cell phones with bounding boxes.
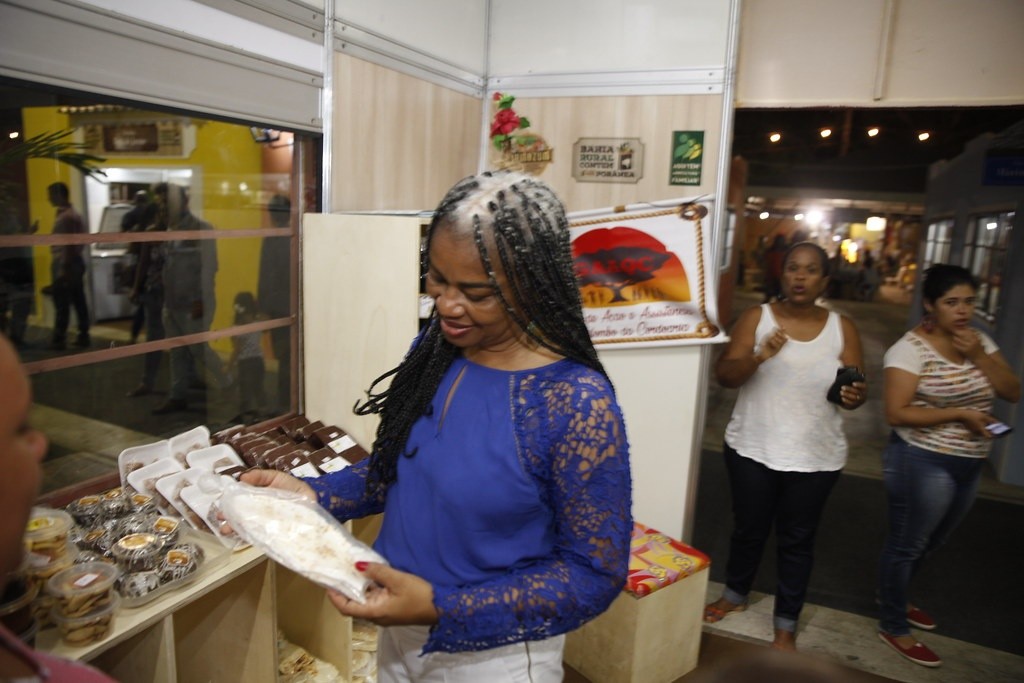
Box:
[984,419,1014,440]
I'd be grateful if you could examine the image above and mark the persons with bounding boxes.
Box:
[0,332,121,683]
[44,183,91,351]
[216,165,634,683]
[0,178,41,351]
[221,291,272,422]
[876,262,1023,668]
[753,231,879,306]
[113,182,218,425]
[701,244,867,653]
[254,193,292,414]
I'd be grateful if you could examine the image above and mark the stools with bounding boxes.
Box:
[561,522,712,683]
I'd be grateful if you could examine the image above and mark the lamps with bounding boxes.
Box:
[867,126,881,137]
[768,131,783,145]
[794,213,804,221]
[759,211,770,220]
[916,129,932,144]
[866,216,888,231]
[819,127,833,139]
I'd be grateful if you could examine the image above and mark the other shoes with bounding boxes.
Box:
[875,626,945,668]
[902,604,936,631]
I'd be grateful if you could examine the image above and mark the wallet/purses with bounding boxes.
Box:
[825,366,865,407]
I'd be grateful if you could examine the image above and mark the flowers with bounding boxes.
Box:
[489,92,531,153]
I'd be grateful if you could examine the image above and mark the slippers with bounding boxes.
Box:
[700,598,744,623]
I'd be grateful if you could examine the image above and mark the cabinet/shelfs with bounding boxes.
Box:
[35,517,353,683]
